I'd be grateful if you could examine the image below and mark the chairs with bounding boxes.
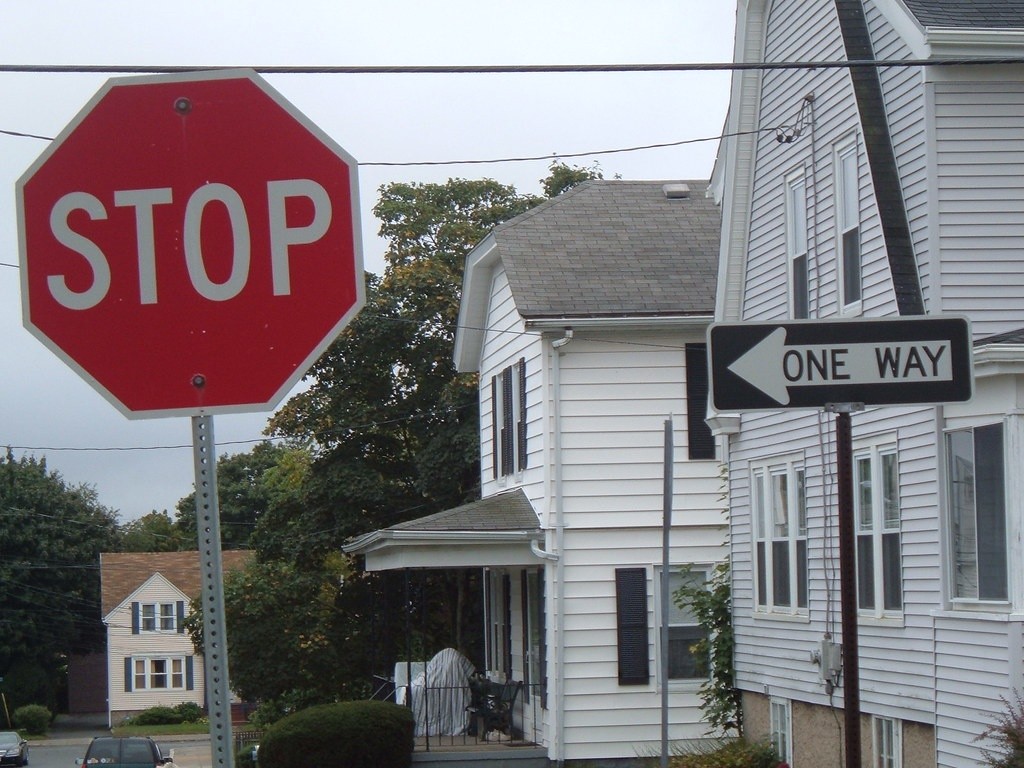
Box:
[464,678,523,743]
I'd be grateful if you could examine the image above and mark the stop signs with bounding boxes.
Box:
[16,69,364,420]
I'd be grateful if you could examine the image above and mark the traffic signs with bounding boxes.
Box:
[705,312,974,414]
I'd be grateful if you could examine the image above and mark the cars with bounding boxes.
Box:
[0,731,30,767]
[74,736,174,768]
